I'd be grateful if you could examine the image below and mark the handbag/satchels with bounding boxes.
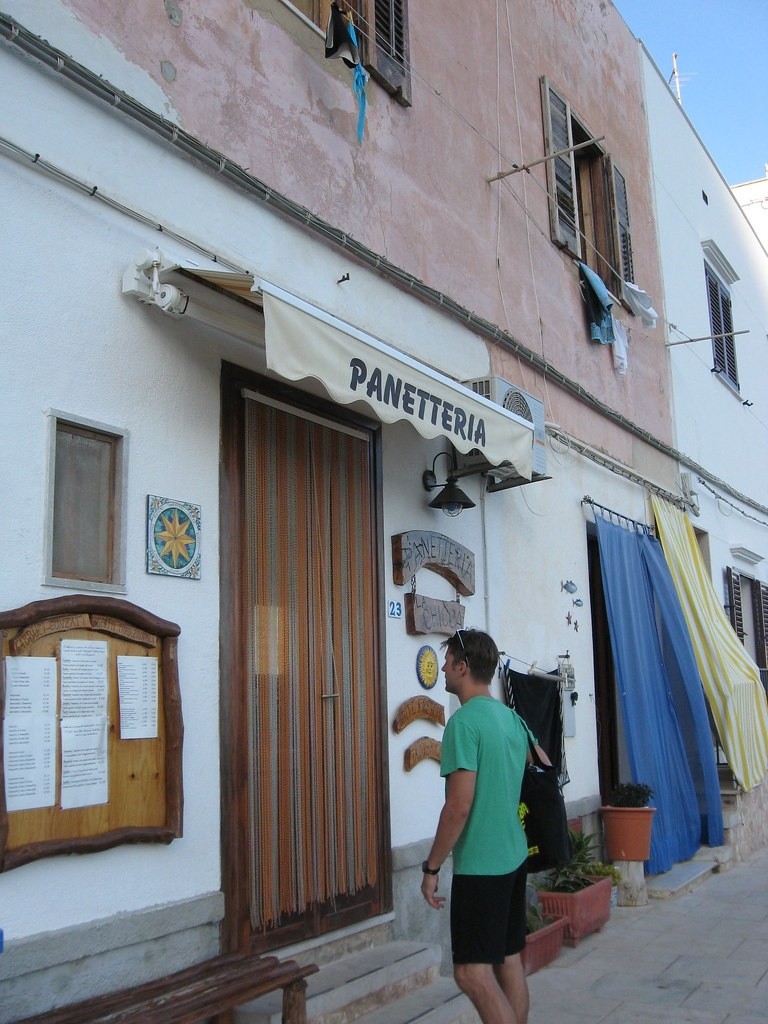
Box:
[517,718,574,874]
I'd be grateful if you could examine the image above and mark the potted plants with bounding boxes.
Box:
[585,862,624,911]
[518,900,569,976]
[530,825,613,948]
[601,781,657,861]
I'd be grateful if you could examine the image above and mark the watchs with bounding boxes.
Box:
[422,860,441,875]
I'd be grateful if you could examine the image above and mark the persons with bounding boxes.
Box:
[420,628,553,1024]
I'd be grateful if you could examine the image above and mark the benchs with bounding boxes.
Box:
[14,951,320,1024]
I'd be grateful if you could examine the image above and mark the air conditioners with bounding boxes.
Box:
[458,374,547,480]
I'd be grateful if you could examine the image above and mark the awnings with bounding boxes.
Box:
[185,266,536,483]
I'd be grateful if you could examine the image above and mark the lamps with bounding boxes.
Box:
[424,452,477,517]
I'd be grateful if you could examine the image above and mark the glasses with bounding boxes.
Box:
[452,628,469,667]
